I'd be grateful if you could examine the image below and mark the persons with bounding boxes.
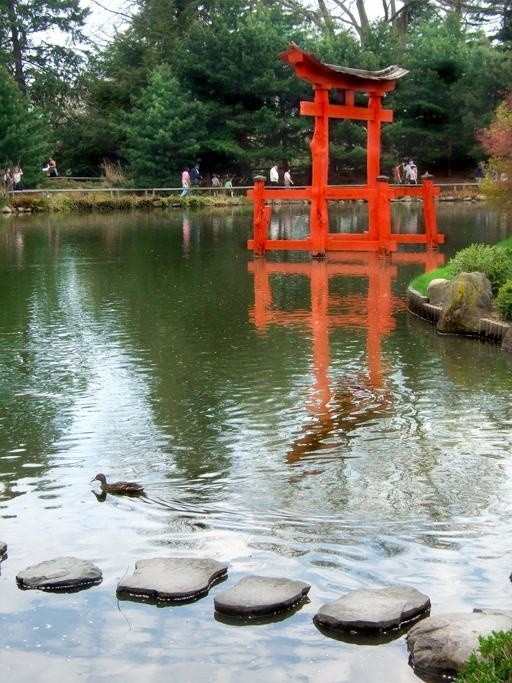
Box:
[190,162,203,196]
[46,157,59,177]
[283,167,294,190]
[224,178,233,197]
[1,168,12,187]
[211,173,221,197]
[269,163,279,190]
[12,167,26,190]
[180,166,191,197]
[391,158,417,184]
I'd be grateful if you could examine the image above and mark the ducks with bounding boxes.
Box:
[90,473,145,492]
[89,490,108,503]
